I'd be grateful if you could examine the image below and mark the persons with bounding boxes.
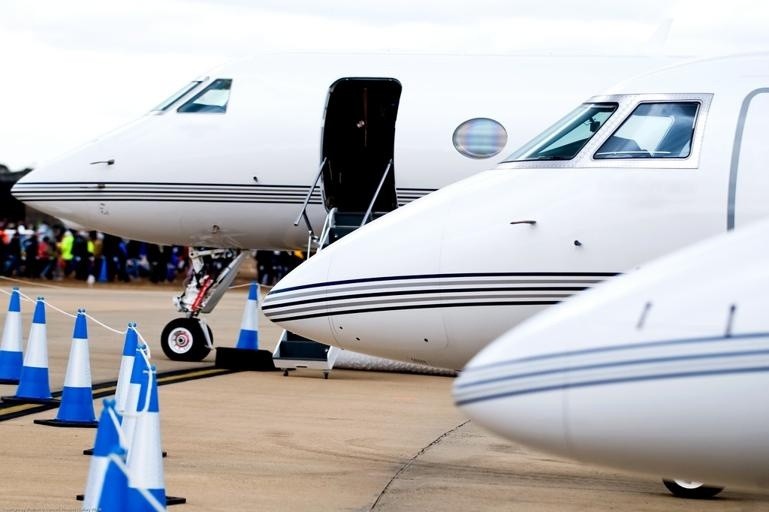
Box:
[253,250,306,285]
[0,221,186,284]
